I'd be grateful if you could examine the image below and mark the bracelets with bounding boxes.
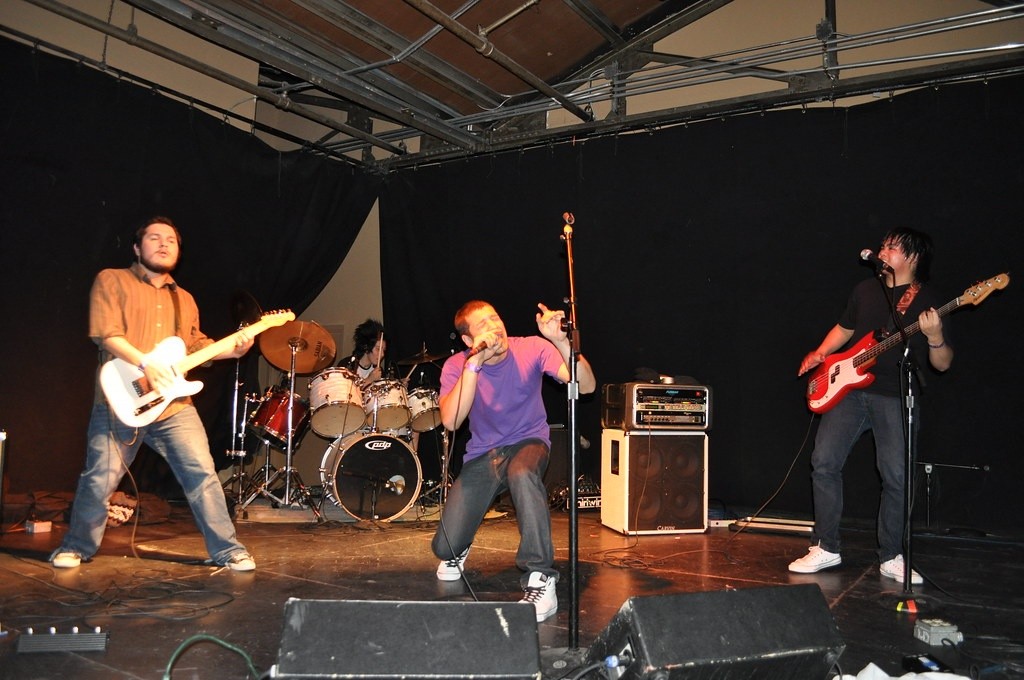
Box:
[138,364,145,370]
[464,362,482,373]
[929,341,945,348]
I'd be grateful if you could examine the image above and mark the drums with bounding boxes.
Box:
[383,424,412,438]
[307,366,367,438]
[320,431,423,523]
[362,378,413,431]
[249,384,311,455]
[407,386,443,433]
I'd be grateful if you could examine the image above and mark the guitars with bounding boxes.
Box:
[98,309,296,428]
[806,272,1010,414]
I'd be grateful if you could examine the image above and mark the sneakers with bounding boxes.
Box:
[516,570,558,624]
[52,548,82,568]
[436,548,470,581]
[879,554,923,585]
[225,553,256,572]
[788,539,841,574]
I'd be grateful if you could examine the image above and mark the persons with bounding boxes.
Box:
[337,319,418,452]
[789,226,956,585]
[431,300,597,621]
[50,216,257,571]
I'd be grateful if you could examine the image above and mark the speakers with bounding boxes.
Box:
[270,596,545,680]
[581,580,846,680]
[597,430,708,536]
[499,429,579,505]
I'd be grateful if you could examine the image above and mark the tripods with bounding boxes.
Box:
[418,426,454,506]
[222,344,326,525]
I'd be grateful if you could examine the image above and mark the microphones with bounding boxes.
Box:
[385,363,392,380]
[347,351,356,371]
[466,336,499,360]
[388,479,405,494]
[860,248,895,272]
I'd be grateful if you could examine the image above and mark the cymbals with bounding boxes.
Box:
[259,321,336,374]
[397,352,452,365]
